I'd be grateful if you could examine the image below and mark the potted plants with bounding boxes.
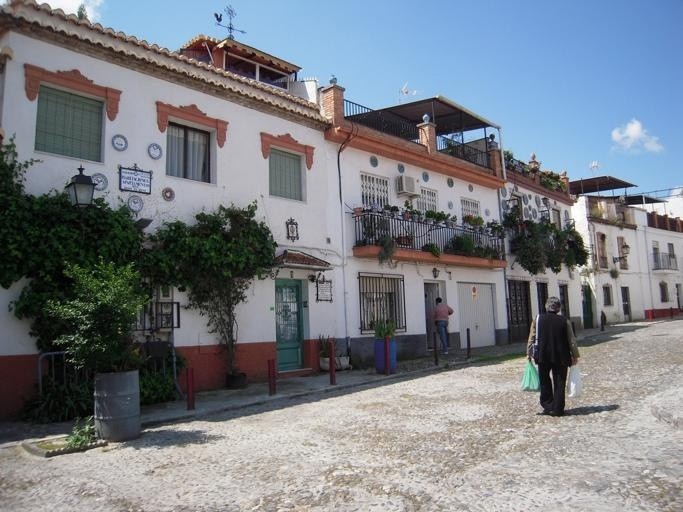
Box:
[316,332,338,371]
[371,318,399,376]
[335,351,351,371]
[86,336,147,444]
[352,203,505,249]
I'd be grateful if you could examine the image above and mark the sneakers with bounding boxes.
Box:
[542,408,565,416]
[440,352,449,355]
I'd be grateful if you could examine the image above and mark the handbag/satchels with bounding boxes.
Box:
[529,314,540,361]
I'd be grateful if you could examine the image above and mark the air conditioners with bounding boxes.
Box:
[394,172,423,200]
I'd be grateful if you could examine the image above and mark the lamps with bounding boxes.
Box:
[64,163,97,208]
[613,241,631,264]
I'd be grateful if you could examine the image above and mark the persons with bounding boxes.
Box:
[525,295,578,416]
[433,297,453,355]
